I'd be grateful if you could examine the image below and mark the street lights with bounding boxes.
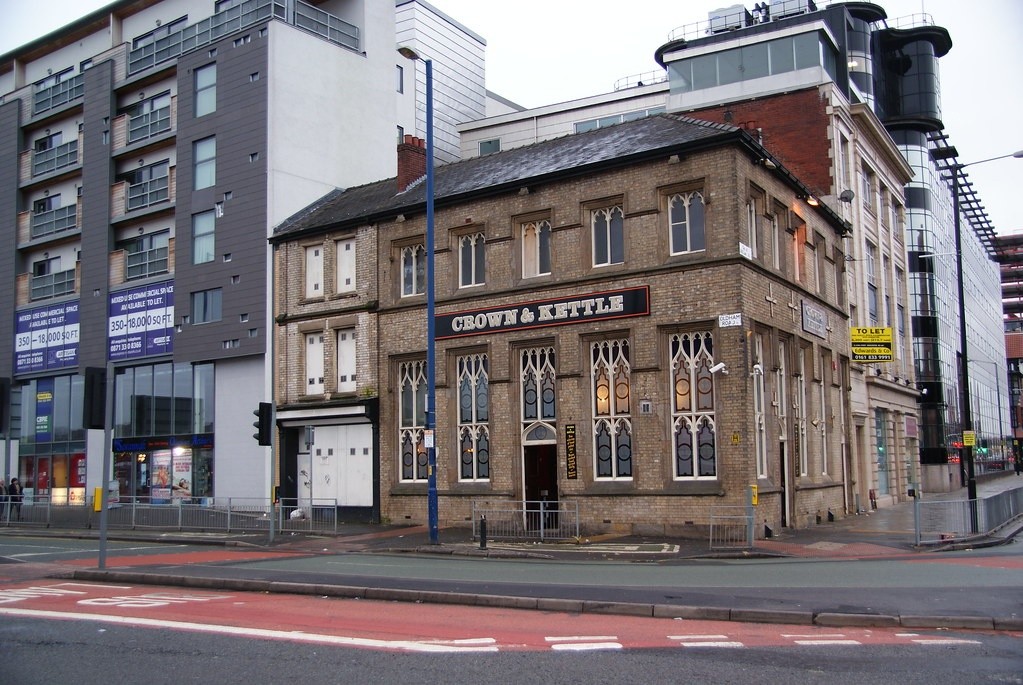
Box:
[397,43,440,549]
[967,358,1006,470]
[951,150,1023,536]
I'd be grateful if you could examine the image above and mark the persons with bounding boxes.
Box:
[0,478,25,522]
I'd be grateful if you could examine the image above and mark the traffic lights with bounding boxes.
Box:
[252,402,270,446]
